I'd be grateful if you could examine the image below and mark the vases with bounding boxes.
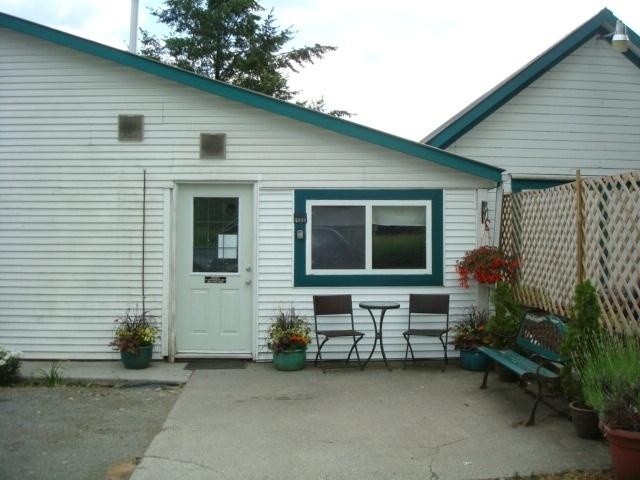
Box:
[460,347,490,371]
[119,343,154,369]
[273,345,308,371]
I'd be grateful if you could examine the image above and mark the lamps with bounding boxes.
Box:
[596,20,630,54]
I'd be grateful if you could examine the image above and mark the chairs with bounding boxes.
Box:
[403,293,451,374]
[312,294,365,374]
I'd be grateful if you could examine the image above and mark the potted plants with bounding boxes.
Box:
[566,276,619,437]
[575,329,640,480]
[485,283,527,383]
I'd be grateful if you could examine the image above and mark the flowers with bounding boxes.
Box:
[448,315,485,350]
[456,243,519,287]
[263,303,313,349]
[110,306,163,346]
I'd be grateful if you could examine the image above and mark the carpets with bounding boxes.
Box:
[183,359,249,371]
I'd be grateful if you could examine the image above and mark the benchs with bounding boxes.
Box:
[477,310,579,430]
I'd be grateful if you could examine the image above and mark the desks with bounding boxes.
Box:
[358,301,401,372]
